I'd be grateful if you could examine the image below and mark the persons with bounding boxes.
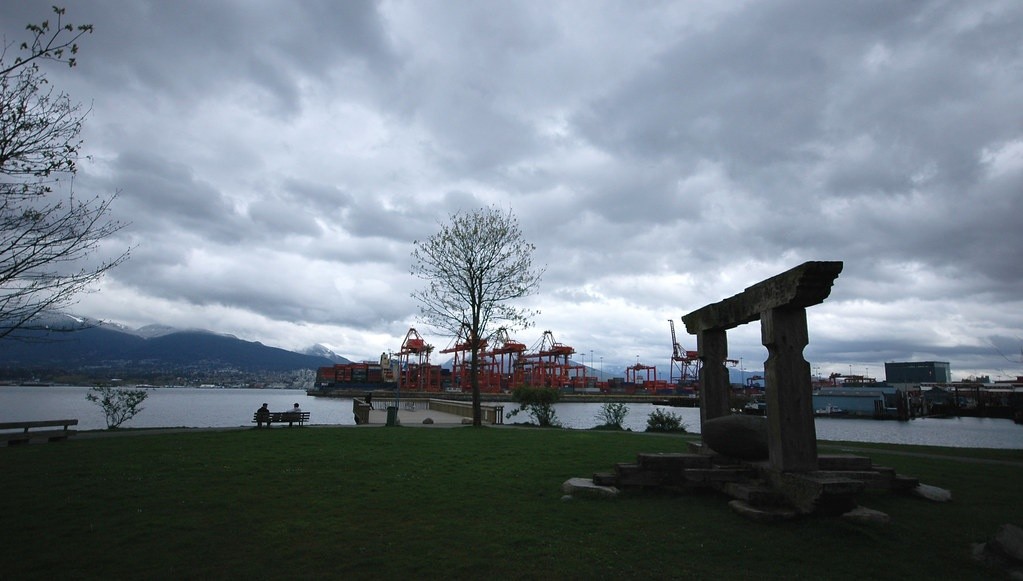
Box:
[365,393,374,410]
[287,404,301,427]
[257,403,271,428]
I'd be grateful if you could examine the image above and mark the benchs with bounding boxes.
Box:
[0,419,78,446]
[251,412,310,429]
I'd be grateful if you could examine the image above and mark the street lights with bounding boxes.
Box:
[849,364,851,374]
[813,366,821,376]
[591,350,594,377]
[580,353,587,366]
[600,356,603,382]
[866,368,869,379]
[740,357,744,384]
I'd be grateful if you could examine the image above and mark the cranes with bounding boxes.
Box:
[394,326,435,393]
[439,320,587,395]
[667,318,703,385]
